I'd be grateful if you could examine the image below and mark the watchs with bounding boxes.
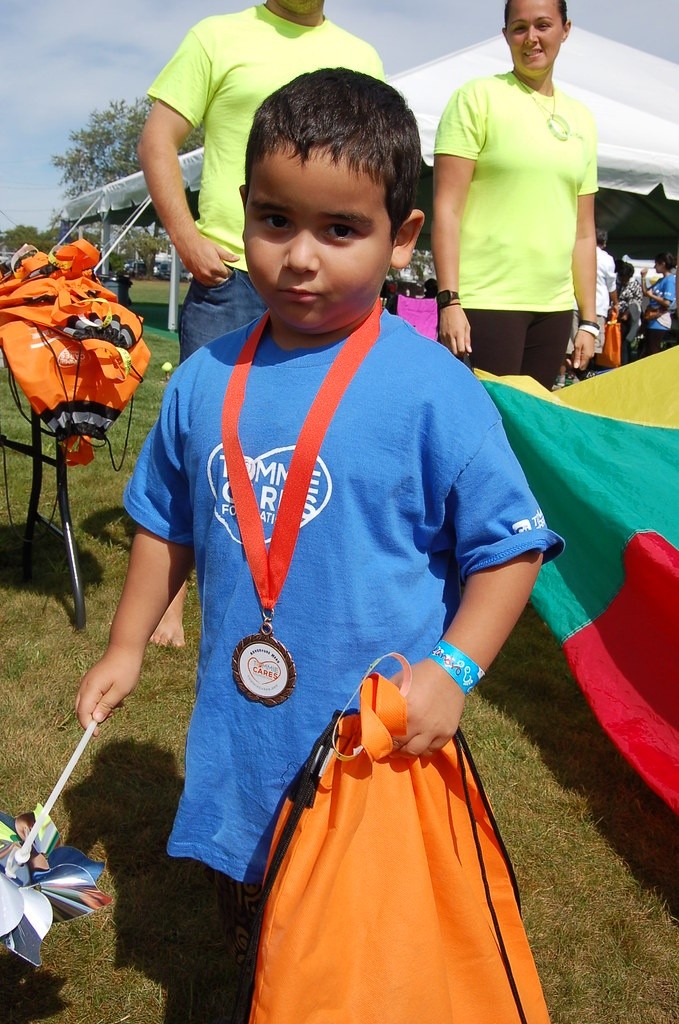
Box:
[435,289,461,306]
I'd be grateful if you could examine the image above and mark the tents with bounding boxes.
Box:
[64,23,679,334]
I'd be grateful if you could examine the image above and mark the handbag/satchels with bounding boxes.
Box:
[593,309,620,368]
[640,307,667,322]
[245,671,552,1024]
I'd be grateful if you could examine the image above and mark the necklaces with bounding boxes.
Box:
[511,72,571,142]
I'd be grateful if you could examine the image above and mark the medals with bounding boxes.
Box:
[232,633,297,708]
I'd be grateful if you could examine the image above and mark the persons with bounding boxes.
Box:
[430,0,600,392]
[135,0,385,366]
[73,66,566,978]
[552,228,676,391]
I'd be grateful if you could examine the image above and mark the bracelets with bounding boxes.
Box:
[439,303,461,309]
[426,639,486,696]
[578,320,600,338]
